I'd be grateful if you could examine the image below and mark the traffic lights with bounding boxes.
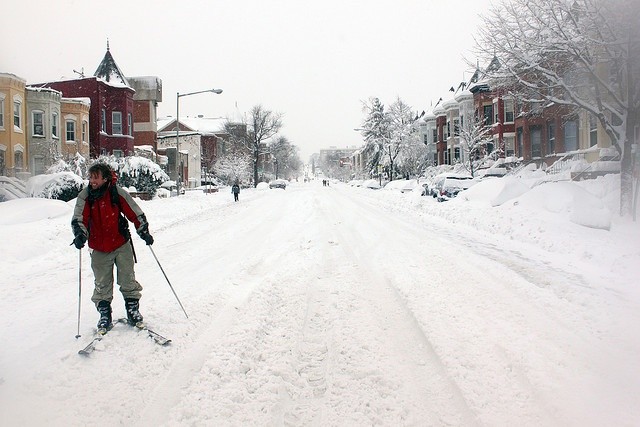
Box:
[340,159,343,166]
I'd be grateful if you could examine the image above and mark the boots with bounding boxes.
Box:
[97,300,112,327]
[125,299,143,322]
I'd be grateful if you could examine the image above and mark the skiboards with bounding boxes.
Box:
[79,317,172,354]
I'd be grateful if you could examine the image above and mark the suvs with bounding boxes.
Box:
[269,180,286,189]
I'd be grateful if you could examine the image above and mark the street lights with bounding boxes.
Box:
[175,89,222,195]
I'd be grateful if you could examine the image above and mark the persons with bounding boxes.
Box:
[71,163,154,330]
[231,182,240,202]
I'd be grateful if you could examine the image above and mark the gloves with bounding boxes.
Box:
[73,232,87,249]
[139,228,154,245]
